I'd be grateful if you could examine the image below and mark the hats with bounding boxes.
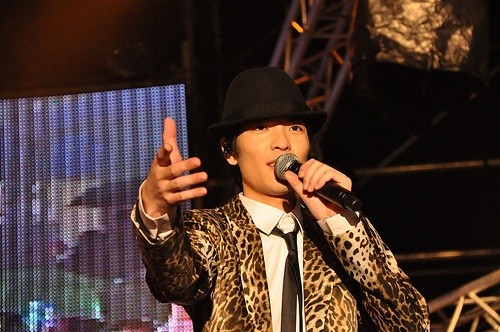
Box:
[206,67,328,135]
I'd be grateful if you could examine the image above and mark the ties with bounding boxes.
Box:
[271,216,304,332]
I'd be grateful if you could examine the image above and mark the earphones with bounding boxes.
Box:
[224,142,233,154]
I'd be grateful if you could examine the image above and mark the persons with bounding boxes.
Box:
[130,66,431,332]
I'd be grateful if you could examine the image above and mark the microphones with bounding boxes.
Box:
[275,153,362,212]
